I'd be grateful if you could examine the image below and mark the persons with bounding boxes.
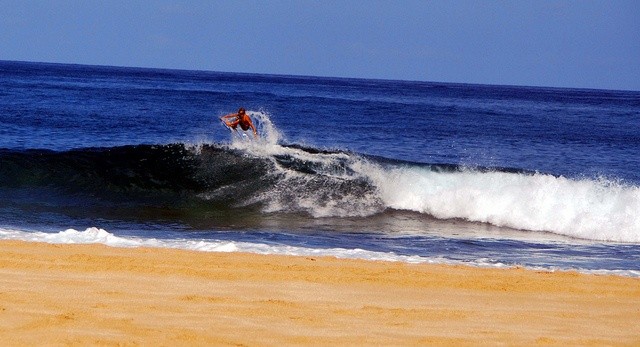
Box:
[221,108,256,137]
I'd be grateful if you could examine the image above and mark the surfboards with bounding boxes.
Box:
[221,118,244,143]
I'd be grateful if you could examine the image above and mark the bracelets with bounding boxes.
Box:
[254,132,257,134]
